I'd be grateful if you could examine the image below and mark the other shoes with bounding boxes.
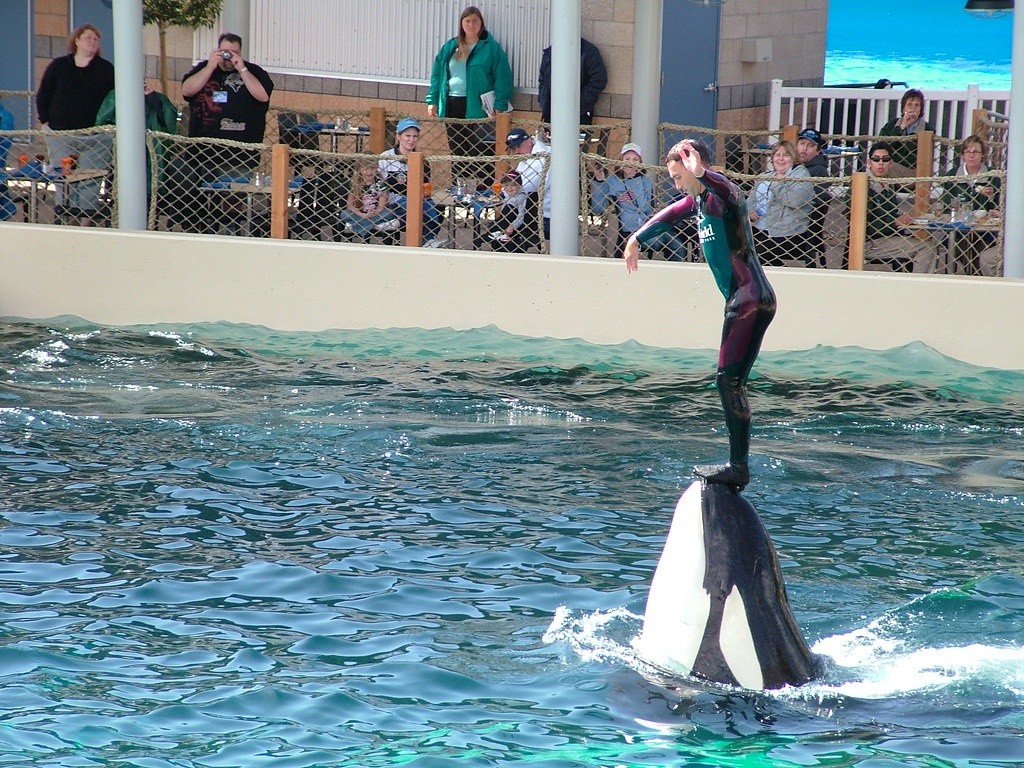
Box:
[378,219,400,230]
[345,222,354,233]
[488,231,509,242]
[423,239,450,248]
[693,462,749,485]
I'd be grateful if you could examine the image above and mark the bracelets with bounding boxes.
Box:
[238,66,248,75]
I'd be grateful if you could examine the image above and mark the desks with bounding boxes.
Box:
[431,190,503,250]
[199,177,302,237]
[897,212,1000,275]
[7,168,109,222]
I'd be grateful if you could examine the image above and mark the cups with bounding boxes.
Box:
[950,198,960,221]
[254,167,265,186]
[933,203,944,219]
[467,179,476,198]
[962,202,973,222]
[457,178,466,196]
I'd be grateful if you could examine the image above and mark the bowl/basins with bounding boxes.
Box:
[974,210,987,218]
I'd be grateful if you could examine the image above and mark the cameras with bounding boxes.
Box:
[220,50,233,60]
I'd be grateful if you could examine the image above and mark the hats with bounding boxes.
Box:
[621,143,642,156]
[501,172,523,185]
[397,118,421,133]
[504,128,530,150]
[798,128,821,146]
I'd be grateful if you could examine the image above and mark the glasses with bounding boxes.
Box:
[870,155,890,162]
[506,133,528,145]
[799,132,818,139]
[963,150,982,155]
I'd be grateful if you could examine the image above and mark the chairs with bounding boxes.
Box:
[778,192,832,268]
[491,192,541,254]
[310,159,357,243]
[187,149,263,236]
[278,113,300,176]
[299,112,320,167]
[335,177,401,246]
[250,175,336,241]
[613,196,670,260]
[285,124,371,176]
[168,159,203,233]
[55,163,114,227]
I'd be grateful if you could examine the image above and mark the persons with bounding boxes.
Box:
[844,141,936,274]
[745,128,832,267]
[979,208,1003,277]
[590,143,686,262]
[940,135,1001,276]
[94,72,178,230]
[539,37,608,168]
[621,137,778,487]
[425,6,514,190]
[339,118,447,249]
[181,32,274,231]
[36,24,114,225]
[877,90,935,188]
[478,128,551,254]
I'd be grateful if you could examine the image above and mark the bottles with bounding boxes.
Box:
[70,155,79,170]
[61,158,71,175]
[424,183,432,195]
[36,155,45,162]
[18,155,28,168]
[493,178,502,195]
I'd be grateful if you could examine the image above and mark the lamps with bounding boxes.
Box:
[963,0,1015,20]
